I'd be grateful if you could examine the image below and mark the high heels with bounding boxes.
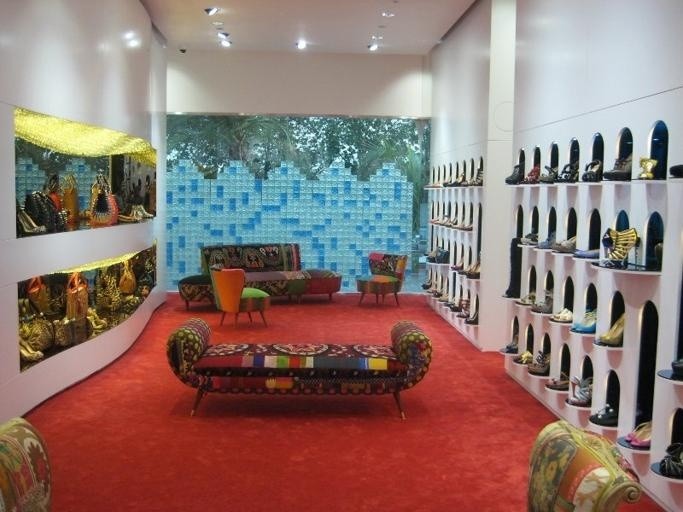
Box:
[17,210,46,235]
[592,229,638,269]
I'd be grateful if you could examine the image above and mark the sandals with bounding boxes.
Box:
[506,158,661,183]
[443,169,483,187]
[516,292,572,322]
[625,421,683,479]
[506,335,616,426]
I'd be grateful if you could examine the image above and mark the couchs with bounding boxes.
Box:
[178,243,341,311]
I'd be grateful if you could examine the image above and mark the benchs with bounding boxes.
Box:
[167,317,432,421]
[0,416,642,512]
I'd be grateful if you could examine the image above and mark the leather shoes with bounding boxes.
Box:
[422,279,477,324]
[450,260,480,278]
[430,218,472,231]
[569,309,624,347]
[521,234,599,258]
[18,336,44,361]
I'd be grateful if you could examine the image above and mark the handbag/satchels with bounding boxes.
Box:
[119,261,136,293]
[18,299,54,351]
[96,272,122,311]
[28,276,47,300]
[26,173,79,232]
[67,273,89,320]
[90,174,118,225]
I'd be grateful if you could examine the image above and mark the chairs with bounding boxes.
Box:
[357,252,407,307]
[209,265,270,329]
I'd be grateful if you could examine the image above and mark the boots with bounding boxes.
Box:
[502,237,521,298]
[130,205,153,218]
